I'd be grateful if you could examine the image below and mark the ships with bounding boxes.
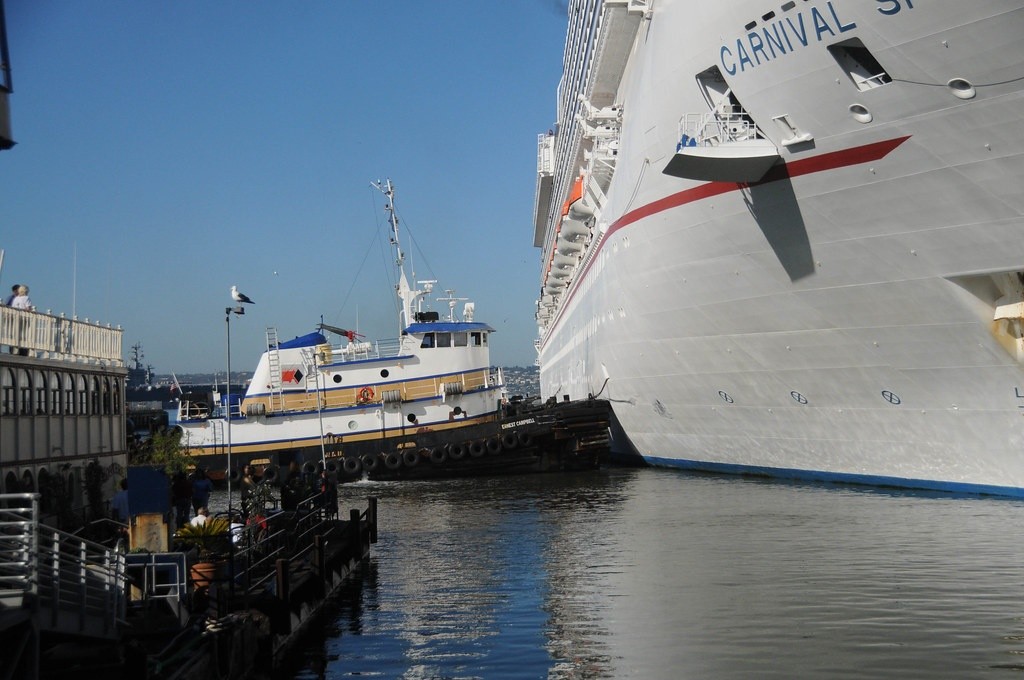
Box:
[529,3,1024,502]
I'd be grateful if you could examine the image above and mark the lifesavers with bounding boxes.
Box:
[359,386,374,402]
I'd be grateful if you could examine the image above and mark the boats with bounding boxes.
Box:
[120,341,164,436]
[164,177,613,483]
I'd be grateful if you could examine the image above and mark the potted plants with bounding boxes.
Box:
[173,515,232,594]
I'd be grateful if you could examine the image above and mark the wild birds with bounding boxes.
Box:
[230,285,255,306]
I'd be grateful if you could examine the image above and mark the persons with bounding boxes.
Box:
[228,515,245,544]
[191,468,214,518]
[6,285,20,308]
[111,478,129,528]
[190,507,211,529]
[246,510,268,531]
[172,471,194,529]
[10,284,32,356]
[300,462,338,524]
